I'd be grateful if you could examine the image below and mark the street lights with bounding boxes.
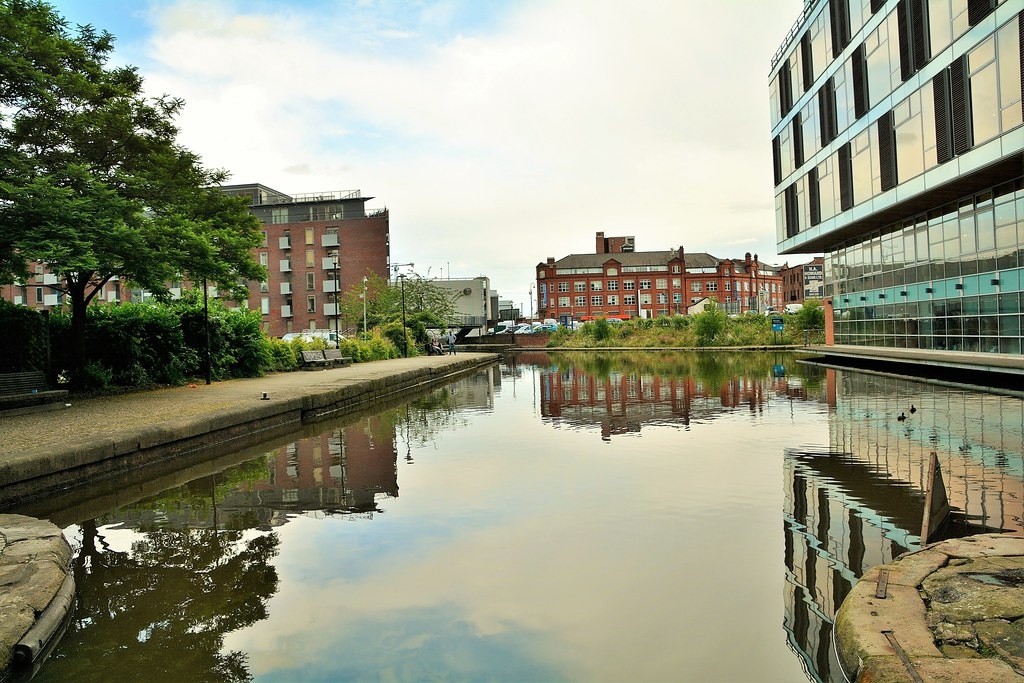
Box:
[401,273,409,357]
[416,291,425,312]
[403,403,414,465]
[362,275,368,342]
[331,428,353,515]
[764,263,779,290]
[529,282,537,333]
[447,262,450,282]
[440,267,442,281]
[331,251,341,345]
[662,291,668,315]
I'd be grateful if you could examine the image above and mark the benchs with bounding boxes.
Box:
[0,371,69,417]
[297,350,335,371]
[323,349,353,369]
[425,343,446,356]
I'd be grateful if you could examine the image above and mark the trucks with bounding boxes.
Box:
[785,304,804,313]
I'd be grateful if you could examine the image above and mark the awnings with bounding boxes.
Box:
[580,315,631,320]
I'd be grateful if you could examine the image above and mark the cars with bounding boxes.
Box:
[495,326,521,334]
[747,309,758,315]
[764,308,781,316]
[533,324,558,333]
[514,325,531,334]
[782,309,795,315]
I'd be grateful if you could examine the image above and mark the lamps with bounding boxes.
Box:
[955,284,962,289]
[828,301,832,304]
[844,299,849,302]
[879,294,885,299]
[900,291,906,296]
[861,297,865,300]
[925,288,932,293]
[991,279,998,285]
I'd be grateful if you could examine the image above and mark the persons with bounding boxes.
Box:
[446,330,457,356]
[432,337,445,355]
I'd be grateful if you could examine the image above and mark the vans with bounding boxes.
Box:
[281,332,350,348]
